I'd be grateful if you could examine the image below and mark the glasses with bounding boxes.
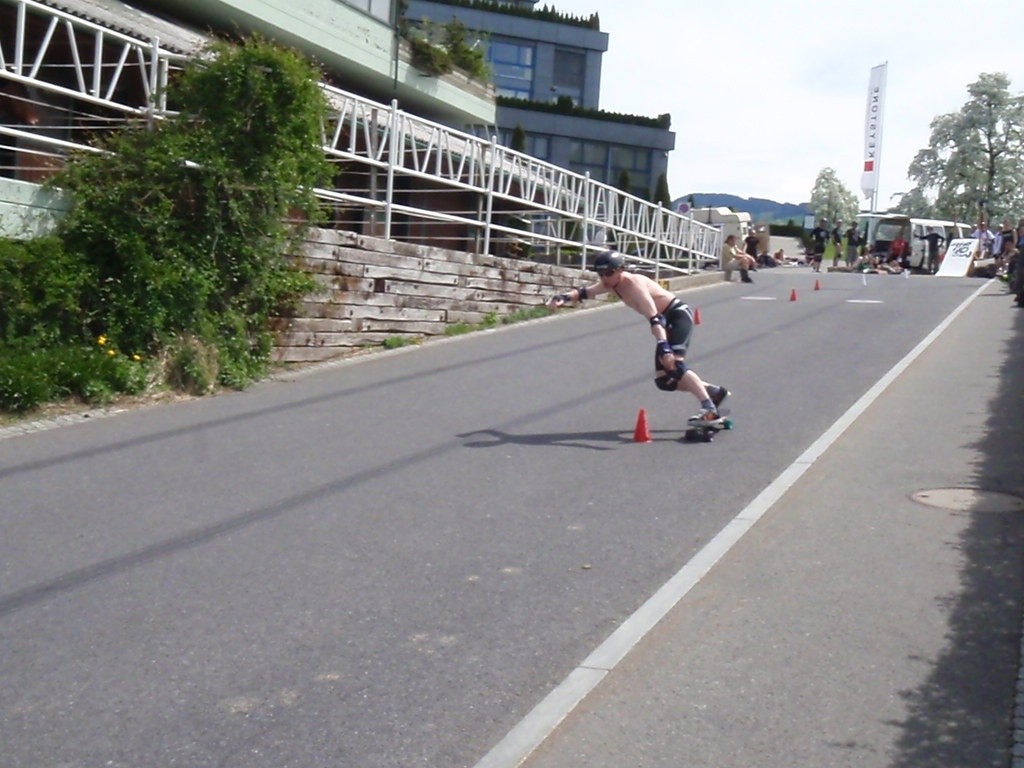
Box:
[598,268,615,277]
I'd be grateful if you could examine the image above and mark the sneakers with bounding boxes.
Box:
[709,386,731,409]
[687,409,727,429]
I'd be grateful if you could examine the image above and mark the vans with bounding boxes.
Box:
[856,213,975,274]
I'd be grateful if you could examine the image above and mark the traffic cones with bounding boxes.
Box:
[791,288,796,301]
[814,279,821,290]
[631,409,651,443]
[693,308,703,324]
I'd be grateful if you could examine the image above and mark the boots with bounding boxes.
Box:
[740,269,752,284]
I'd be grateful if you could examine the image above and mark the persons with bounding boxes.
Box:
[745,229,784,268]
[551,252,731,425]
[832,220,845,267]
[863,240,911,270]
[811,219,840,272]
[970,218,1024,308]
[846,221,860,268]
[722,235,758,283]
[914,227,945,274]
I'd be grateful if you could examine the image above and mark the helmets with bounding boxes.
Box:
[588,251,624,272]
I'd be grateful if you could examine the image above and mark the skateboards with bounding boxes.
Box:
[686,408,734,442]
